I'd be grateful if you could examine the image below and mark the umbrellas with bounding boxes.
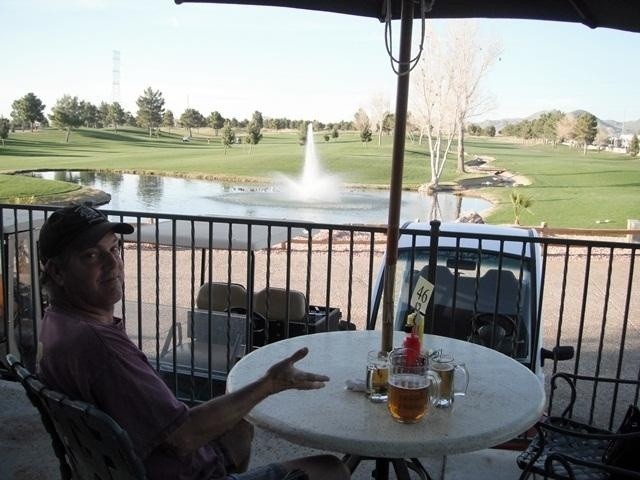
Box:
[173,0,640,359]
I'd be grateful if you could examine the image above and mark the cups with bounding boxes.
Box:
[362,347,470,426]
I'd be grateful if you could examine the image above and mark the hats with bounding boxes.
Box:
[38,203,134,265]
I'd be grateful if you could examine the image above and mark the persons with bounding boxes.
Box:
[33,202,351,480]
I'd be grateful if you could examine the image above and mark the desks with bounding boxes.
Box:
[226,330,546,479]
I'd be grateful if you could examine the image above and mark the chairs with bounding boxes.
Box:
[516,372,640,479]
[7,353,308,480]
[161,282,306,373]
[410,265,521,340]
